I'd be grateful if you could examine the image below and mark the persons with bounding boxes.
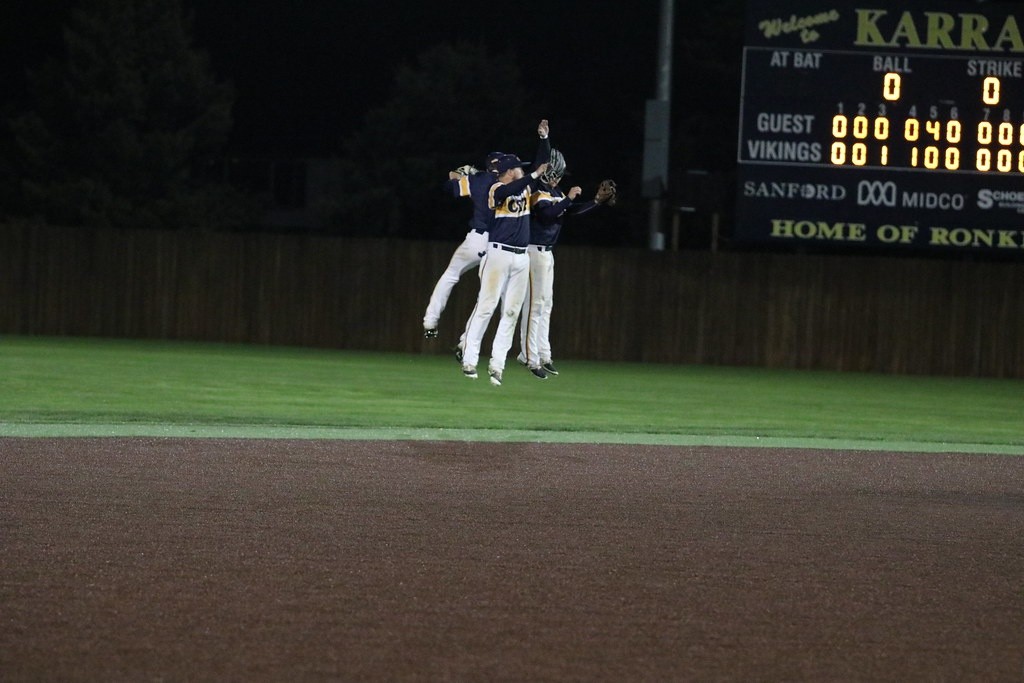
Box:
[518,149,617,379]
[463,119,549,386]
[423,152,506,363]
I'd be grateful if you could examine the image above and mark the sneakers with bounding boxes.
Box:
[528,366,548,379]
[456,345,462,363]
[424,328,439,341]
[542,362,559,375]
[490,372,502,385]
[463,365,478,378]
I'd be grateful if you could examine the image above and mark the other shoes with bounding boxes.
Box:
[517,357,527,366]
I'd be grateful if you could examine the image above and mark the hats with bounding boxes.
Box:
[486,152,506,167]
[496,154,531,173]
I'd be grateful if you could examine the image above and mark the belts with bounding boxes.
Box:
[538,245,553,252]
[476,228,485,235]
[493,243,525,254]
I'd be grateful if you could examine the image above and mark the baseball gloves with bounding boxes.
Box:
[455,165,478,175]
[594,179,618,207]
[540,149,566,184]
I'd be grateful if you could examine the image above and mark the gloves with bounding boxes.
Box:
[478,251,486,257]
